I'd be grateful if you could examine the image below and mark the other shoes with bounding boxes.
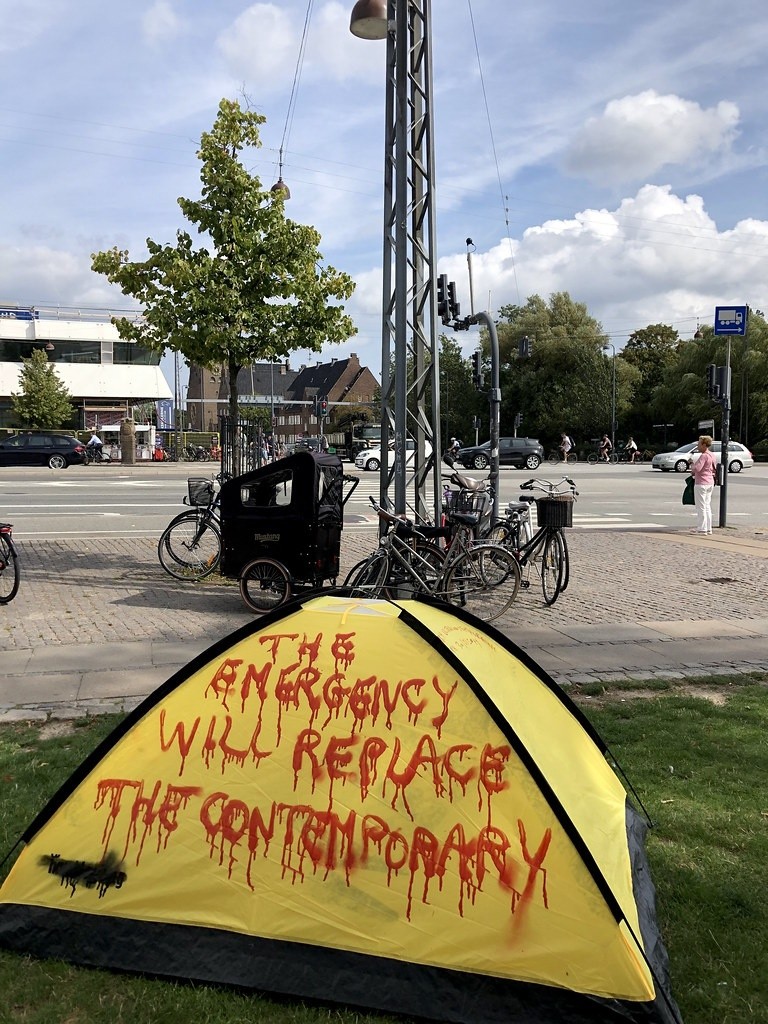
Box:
[630,460,633,462]
[606,457,610,461]
[566,454,570,457]
[562,461,567,463]
[707,529,712,535]
[689,529,707,536]
[600,456,604,459]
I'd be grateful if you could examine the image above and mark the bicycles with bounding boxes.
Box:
[83,447,113,467]
[340,475,581,622]
[548,445,645,465]
[157,451,359,615]
[0,523,23,603]
[160,444,213,462]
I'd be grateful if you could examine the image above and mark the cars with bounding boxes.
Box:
[284,438,321,456]
[355,439,434,472]
[457,438,544,469]
[0,434,86,470]
[651,441,754,474]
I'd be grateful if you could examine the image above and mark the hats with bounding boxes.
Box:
[628,437,633,439]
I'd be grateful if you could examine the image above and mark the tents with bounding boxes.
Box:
[0,585,686,1024]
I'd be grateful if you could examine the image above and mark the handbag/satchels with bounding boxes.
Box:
[682,476,695,505]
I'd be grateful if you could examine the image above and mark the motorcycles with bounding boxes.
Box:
[438,453,499,558]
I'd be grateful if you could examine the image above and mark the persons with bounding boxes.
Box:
[266,435,274,464]
[85,432,104,459]
[447,437,461,459]
[690,435,716,535]
[623,436,638,463]
[561,432,572,462]
[599,434,612,461]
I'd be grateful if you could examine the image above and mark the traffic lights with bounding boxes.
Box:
[471,350,480,385]
[312,395,329,417]
[523,338,532,358]
[446,280,456,315]
[516,412,523,426]
[436,274,450,322]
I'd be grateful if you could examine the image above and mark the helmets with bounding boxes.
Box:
[451,437,456,441]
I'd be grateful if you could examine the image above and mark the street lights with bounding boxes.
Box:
[604,343,616,464]
[440,370,448,451]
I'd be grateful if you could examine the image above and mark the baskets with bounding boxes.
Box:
[188,477,212,506]
[533,499,573,527]
[442,489,486,525]
[539,495,573,500]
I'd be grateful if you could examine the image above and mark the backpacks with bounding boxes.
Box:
[567,436,576,448]
[458,440,464,447]
